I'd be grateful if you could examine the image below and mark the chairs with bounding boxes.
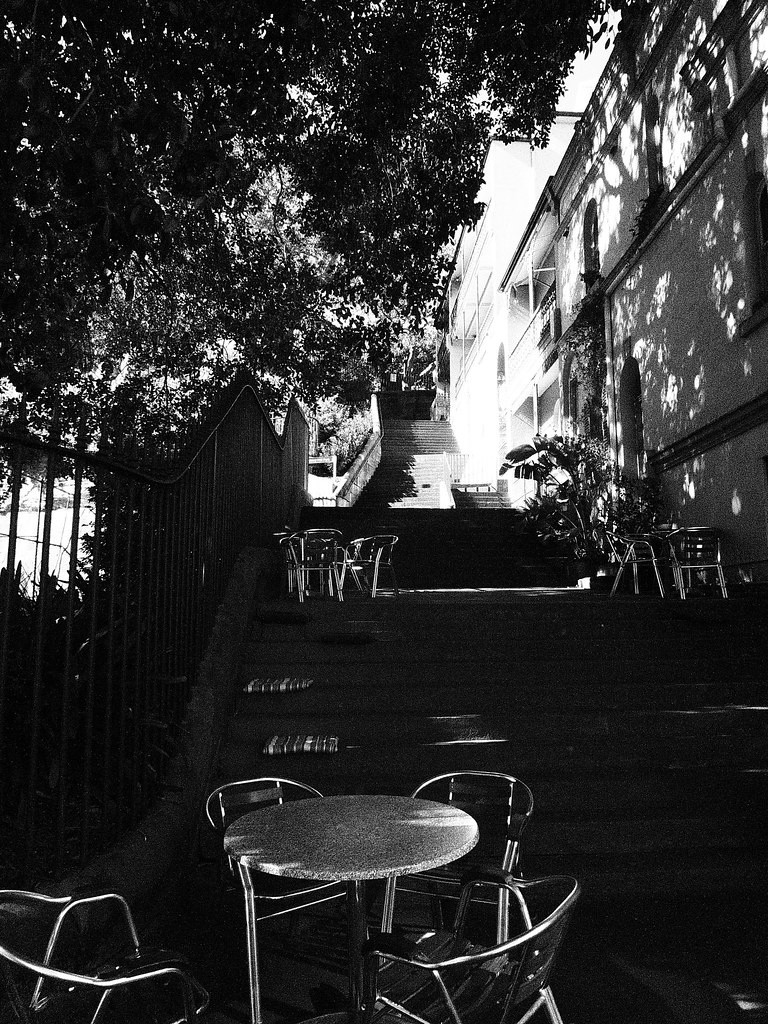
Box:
[362,876,581,1024]
[273,531,324,595]
[284,525,363,592]
[666,526,728,599]
[0,889,210,1024]
[625,532,680,591]
[605,531,666,598]
[205,781,369,943]
[378,769,535,966]
[340,535,401,599]
[288,528,344,602]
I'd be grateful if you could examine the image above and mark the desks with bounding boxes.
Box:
[222,794,479,1024]
[654,526,714,596]
